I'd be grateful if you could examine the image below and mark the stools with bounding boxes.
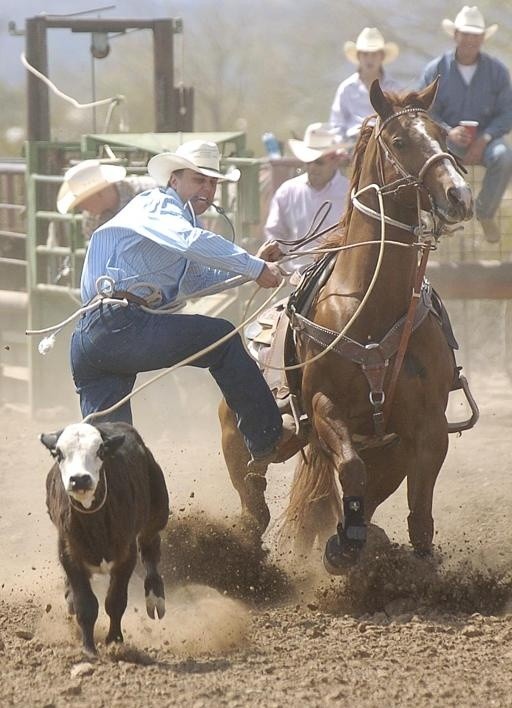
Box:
[438,162,503,262]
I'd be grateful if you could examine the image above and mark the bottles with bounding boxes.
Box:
[261,130,280,159]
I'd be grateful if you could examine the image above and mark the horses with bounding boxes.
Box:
[215,72,475,579]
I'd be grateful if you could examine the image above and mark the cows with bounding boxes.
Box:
[37,419,171,663]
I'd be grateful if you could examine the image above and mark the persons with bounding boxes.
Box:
[415,4,512,242]
[330,25,409,145]
[56,159,153,253]
[263,121,352,270]
[66,141,297,466]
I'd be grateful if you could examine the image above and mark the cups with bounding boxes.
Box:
[458,119,479,142]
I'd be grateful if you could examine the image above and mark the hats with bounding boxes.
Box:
[342,26,399,67]
[145,138,243,190]
[441,5,500,43]
[55,158,128,216]
[287,121,358,164]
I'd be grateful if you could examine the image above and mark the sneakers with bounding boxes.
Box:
[474,211,503,245]
[251,413,296,466]
